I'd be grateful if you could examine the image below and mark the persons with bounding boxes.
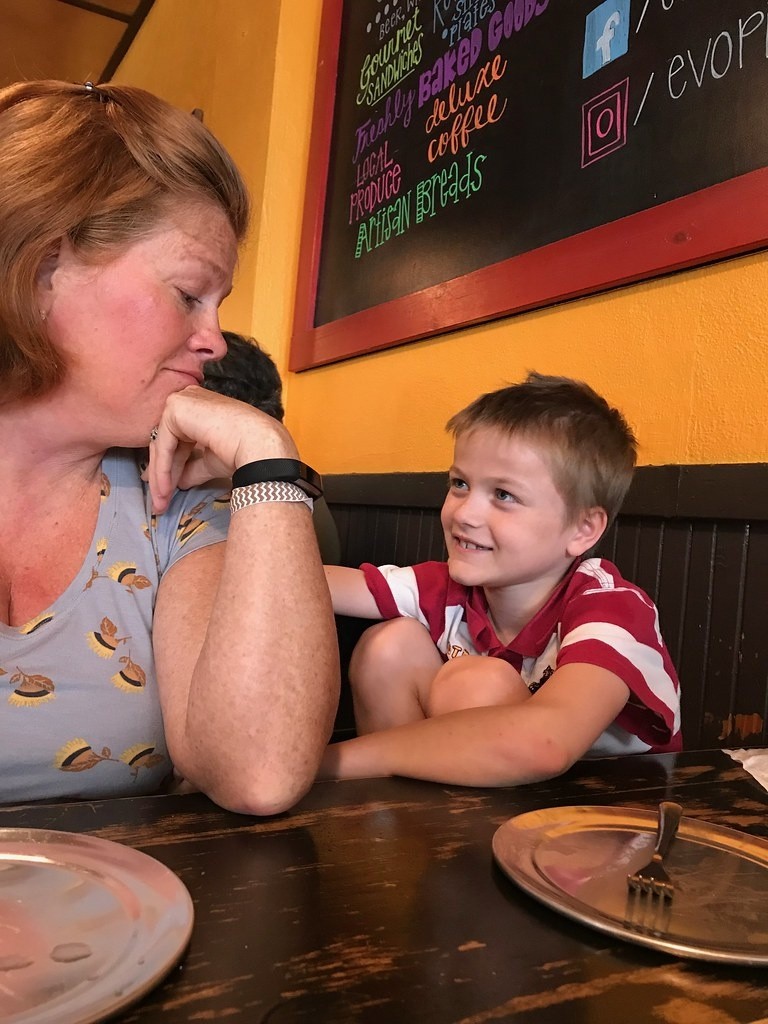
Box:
[201,329,342,745]
[0,76,341,817]
[321,369,684,788]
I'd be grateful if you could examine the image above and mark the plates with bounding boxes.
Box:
[0,825,194,1022]
[492,806,768,969]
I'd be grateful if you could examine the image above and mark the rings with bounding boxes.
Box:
[150,424,159,442]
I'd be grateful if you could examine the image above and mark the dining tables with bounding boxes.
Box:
[0,746,768,1024]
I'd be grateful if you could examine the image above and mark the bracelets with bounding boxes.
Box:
[229,458,324,516]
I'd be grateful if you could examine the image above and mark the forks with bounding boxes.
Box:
[627,801,684,899]
